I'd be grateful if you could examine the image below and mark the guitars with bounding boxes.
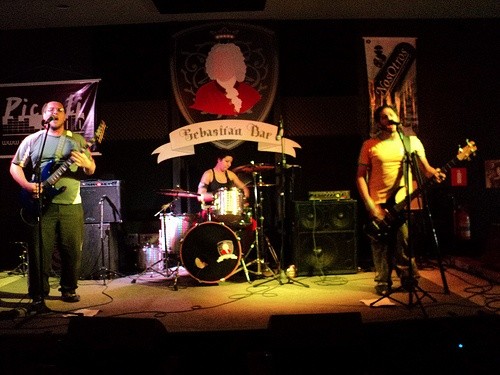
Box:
[365,138,478,243]
[18,119,108,227]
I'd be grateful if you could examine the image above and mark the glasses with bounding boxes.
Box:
[45,108,65,112]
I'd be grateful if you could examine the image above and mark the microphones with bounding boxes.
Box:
[387,119,403,125]
[44,115,56,124]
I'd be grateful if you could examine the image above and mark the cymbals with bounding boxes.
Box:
[154,188,201,198]
[246,182,279,187]
[239,164,275,173]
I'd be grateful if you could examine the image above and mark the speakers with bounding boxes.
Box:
[289,199,360,278]
[66,315,169,375]
[265,312,364,375]
[81,222,119,278]
[78,179,122,223]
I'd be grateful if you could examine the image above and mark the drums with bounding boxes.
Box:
[212,186,244,223]
[159,212,196,255]
[179,221,242,283]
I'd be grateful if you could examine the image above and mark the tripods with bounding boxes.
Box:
[368,128,437,317]
[238,170,310,289]
[13,124,83,328]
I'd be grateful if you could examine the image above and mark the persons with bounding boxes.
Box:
[10,99,96,312]
[197,149,257,245]
[356,105,447,296]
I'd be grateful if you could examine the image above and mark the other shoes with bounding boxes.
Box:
[376,284,391,295]
[402,277,418,290]
[33,300,50,313]
[58,285,79,302]
